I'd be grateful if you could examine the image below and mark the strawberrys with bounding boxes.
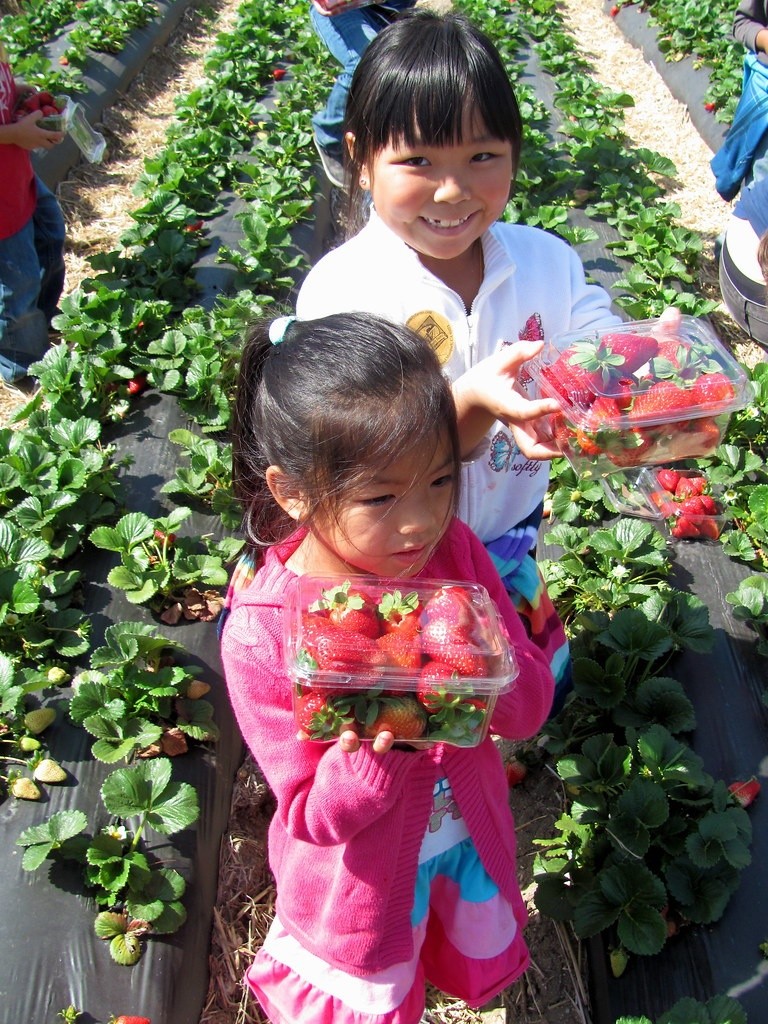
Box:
[24,708,57,733]
[185,220,202,232]
[20,737,41,751]
[34,759,67,781]
[107,1016,151,1024]
[611,5,618,16]
[611,952,628,977]
[651,470,718,539]
[127,375,146,393]
[506,760,525,787]
[187,680,211,699]
[48,667,71,684]
[705,103,714,110]
[273,69,286,80]
[293,583,489,742]
[540,336,735,465]
[11,778,40,799]
[728,782,760,807]
[155,530,175,545]
[14,91,63,117]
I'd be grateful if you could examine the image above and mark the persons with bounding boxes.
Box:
[296,13,681,721]
[709,0,768,346]
[0,38,65,392]
[309,0,417,190]
[221,311,555,1024]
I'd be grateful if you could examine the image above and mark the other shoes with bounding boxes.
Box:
[3,376,39,399]
[313,133,353,189]
